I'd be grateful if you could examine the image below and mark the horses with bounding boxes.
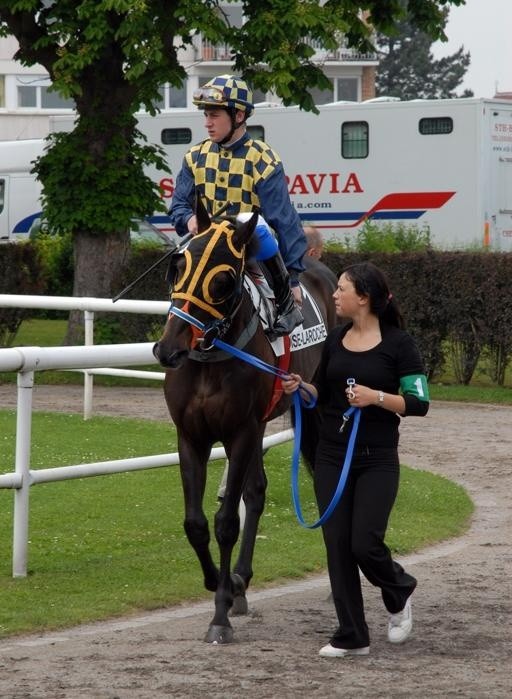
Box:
[151,186,354,646]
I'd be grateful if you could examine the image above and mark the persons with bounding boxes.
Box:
[167,74,309,338]
[293,262,429,658]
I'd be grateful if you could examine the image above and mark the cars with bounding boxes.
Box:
[130,218,176,248]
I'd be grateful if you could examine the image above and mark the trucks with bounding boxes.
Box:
[0,95,512,251]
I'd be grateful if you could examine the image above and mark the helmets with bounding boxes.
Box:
[193,75,254,115]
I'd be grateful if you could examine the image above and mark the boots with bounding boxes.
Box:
[255,251,304,335]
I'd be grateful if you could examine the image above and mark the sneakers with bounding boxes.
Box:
[386,593,413,645]
[319,642,370,657]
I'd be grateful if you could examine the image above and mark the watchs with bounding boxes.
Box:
[377,390,384,407]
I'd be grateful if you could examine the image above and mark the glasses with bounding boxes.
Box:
[193,88,253,106]
